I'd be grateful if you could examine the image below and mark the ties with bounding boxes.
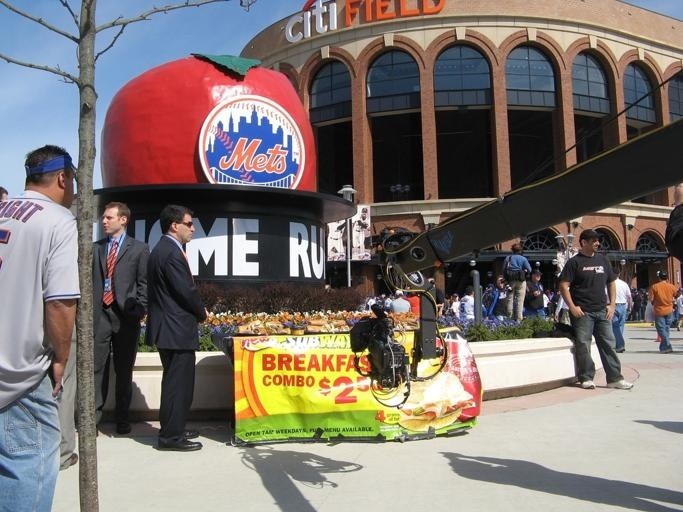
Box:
[102,240,119,306]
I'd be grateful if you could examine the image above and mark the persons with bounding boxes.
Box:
[554,286,573,325]
[605,269,633,353]
[524,269,548,321]
[633,292,642,321]
[677,289,683,330]
[144,205,209,452]
[450,293,461,317]
[0,188,9,204]
[460,285,488,324]
[559,230,635,389]
[648,271,675,353]
[491,275,511,316]
[502,244,532,322]
[92,202,148,435]
[0,145,82,512]
[654,271,663,342]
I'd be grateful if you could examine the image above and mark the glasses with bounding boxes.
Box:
[176,220,193,229]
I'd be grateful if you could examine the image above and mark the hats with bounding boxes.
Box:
[531,268,541,275]
[659,270,668,280]
[24,155,77,176]
[396,288,403,296]
[580,227,606,240]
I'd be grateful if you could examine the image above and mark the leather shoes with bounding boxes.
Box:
[158,430,201,439]
[158,437,203,453]
[116,422,133,435]
[59,453,78,471]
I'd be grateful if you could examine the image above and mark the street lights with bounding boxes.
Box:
[337,185,358,288]
[555,234,575,260]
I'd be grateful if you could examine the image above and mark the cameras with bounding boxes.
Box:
[504,280,513,293]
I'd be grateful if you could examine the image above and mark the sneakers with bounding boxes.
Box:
[607,380,634,390]
[660,346,673,354]
[616,347,626,353]
[581,380,595,389]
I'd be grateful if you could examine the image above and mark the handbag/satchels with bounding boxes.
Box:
[503,258,526,282]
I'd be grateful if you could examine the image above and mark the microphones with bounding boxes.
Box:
[371,303,394,338]
